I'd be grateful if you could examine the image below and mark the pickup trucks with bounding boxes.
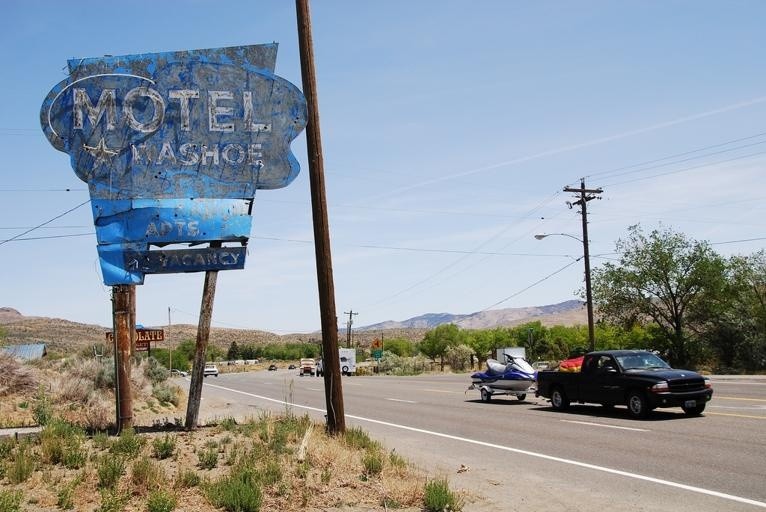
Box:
[535,350,713,416]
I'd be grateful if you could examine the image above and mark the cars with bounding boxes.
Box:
[204,363,218,377]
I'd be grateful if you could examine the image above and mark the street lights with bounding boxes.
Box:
[535,233,595,351]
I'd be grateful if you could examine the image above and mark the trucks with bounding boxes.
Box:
[316,349,357,377]
[300,357,315,376]
